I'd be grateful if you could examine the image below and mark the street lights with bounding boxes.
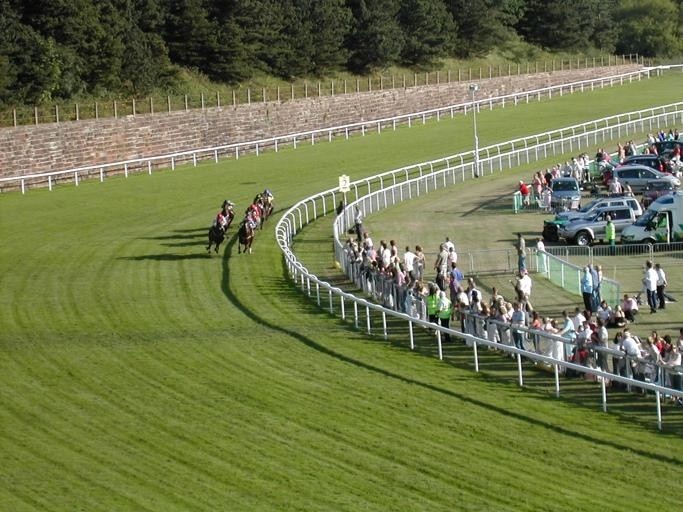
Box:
[470,83,480,177]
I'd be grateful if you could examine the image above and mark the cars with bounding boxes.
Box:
[613,165,680,194]
[603,155,672,186]
[550,177,582,210]
[651,141,683,161]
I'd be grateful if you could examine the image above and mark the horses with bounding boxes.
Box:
[236,191,270,255]
[219,200,236,228]
[204,218,224,256]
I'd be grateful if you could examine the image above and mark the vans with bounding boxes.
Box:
[621,189,683,253]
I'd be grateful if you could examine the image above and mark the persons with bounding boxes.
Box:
[604,214,615,245]
[209,189,273,237]
[457,258,683,402]
[345,205,463,343]
[513,233,526,275]
[655,213,666,228]
[517,129,683,211]
[337,201,344,214]
[535,237,547,254]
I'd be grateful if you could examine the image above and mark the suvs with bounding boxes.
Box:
[556,196,643,216]
[560,205,636,246]
